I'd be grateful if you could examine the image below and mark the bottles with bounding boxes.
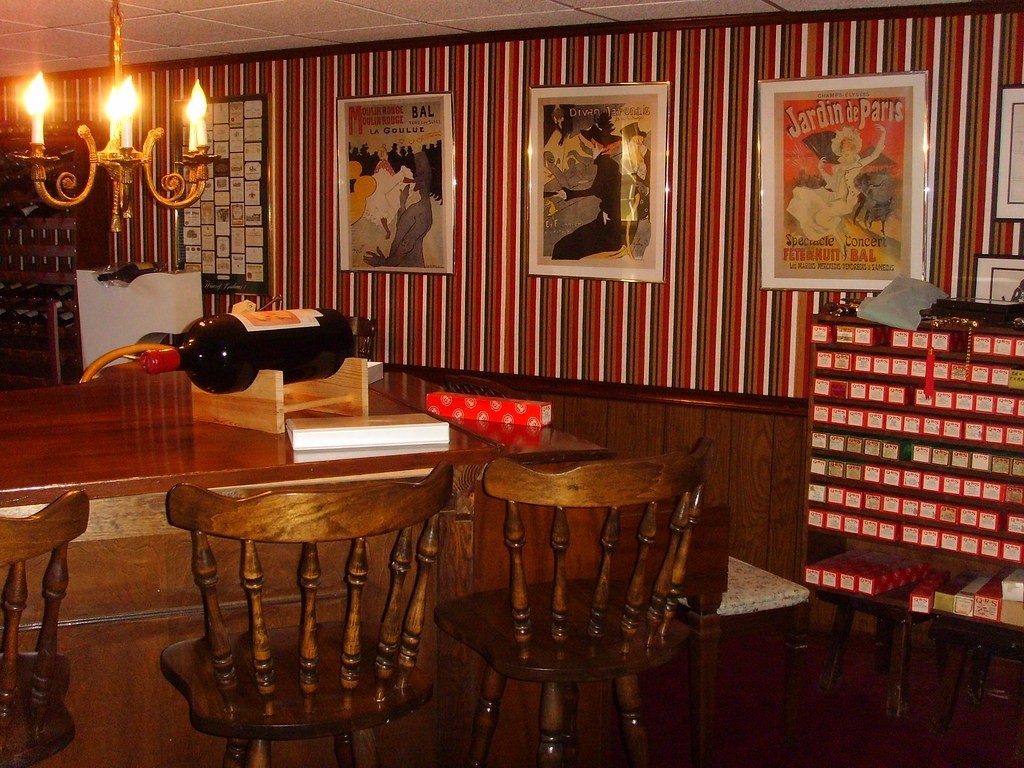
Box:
[0,309,75,337]
[0,201,71,229]
[138,309,354,395]
[0,282,73,309]
[97,261,159,284]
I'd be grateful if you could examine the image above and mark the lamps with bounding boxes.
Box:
[6,0,221,233]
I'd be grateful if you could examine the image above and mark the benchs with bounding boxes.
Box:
[618,502,811,768]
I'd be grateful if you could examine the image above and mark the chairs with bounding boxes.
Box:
[159,461,453,768]
[434,437,714,768]
[0,488,90,768]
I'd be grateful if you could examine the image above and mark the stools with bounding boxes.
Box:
[815,590,937,720]
[929,616,1024,738]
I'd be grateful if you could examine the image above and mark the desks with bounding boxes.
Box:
[1,360,617,767]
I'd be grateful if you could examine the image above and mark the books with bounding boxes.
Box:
[286,413,450,451]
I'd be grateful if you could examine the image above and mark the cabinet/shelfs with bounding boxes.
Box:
[800,311,1024,665]
[0,120,114,386]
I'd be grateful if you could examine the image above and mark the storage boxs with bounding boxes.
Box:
[425,392,553,427]
[803,549,1024,628]
[285,414,449,449]
[368,362,384,385]
[808,323,1024,565]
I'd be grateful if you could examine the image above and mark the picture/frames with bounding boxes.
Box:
[973,254,1024,305]
[992,85,1024,222]
